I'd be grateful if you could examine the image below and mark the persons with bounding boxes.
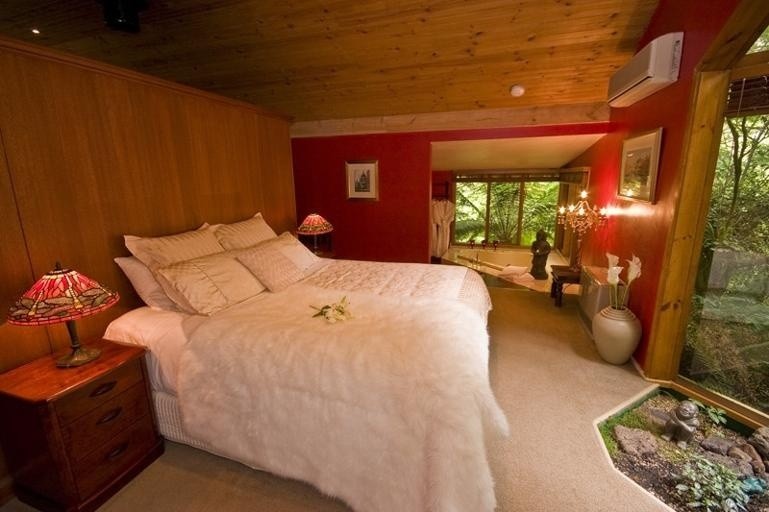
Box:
[660,398,702,452]
[529,229,551,280]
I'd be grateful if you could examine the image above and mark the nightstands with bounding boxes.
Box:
[1,338,168,511]
[314,251,338,260]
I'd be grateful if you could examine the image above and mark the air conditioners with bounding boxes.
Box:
[605,30,685,110]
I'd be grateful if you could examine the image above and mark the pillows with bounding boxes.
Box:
[112,212,324,321]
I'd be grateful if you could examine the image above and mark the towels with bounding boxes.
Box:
[507,273,536,283]
[498,263,530,277]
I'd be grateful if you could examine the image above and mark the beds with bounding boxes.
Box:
[99,210,511,512]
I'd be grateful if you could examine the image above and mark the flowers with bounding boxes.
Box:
[602,251,643,311]
[307,295,354,326]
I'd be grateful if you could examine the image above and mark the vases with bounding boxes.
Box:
[591,303,643,365]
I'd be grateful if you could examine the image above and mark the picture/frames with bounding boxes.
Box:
[343,157,381,202]
[615,126,665,206]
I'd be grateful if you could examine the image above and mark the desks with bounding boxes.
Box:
[549,264,583,308]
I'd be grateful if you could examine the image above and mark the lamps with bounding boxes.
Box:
[556,188,610,272]
[6,260,121,373]
[297,212,335,256]
[509,83,526,99]
